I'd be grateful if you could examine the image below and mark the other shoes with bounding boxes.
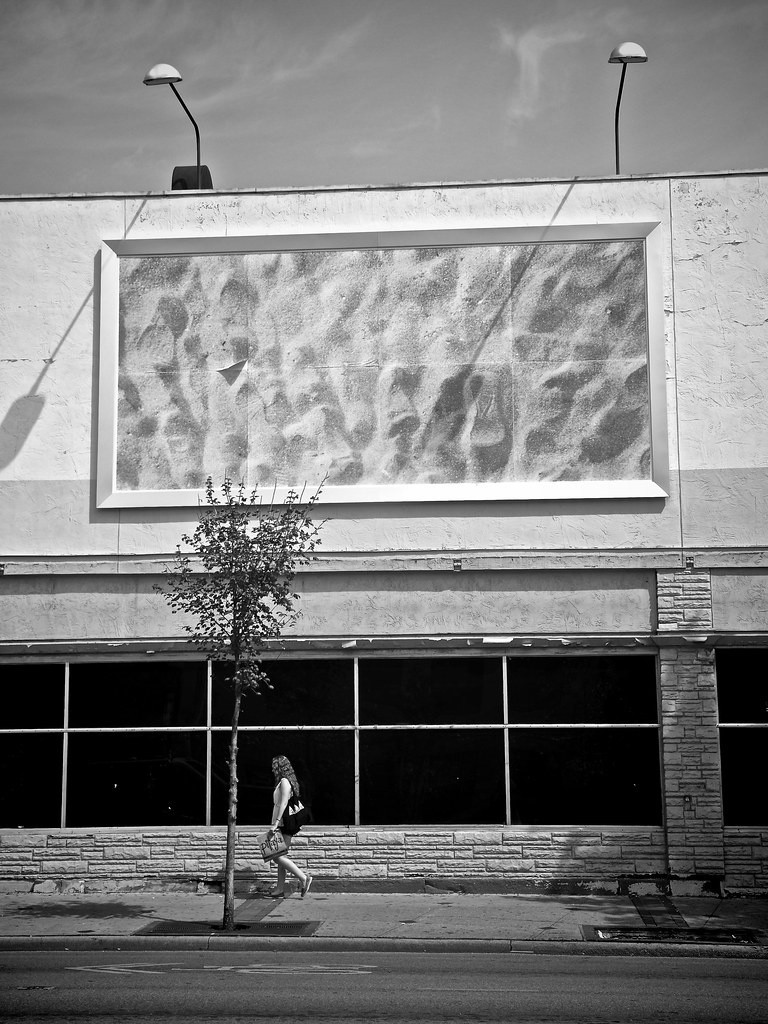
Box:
[301,876,313,897]
[263,891,284,899]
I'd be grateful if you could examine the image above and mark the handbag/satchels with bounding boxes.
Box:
[256,830,288,863]
[280,777,307,830]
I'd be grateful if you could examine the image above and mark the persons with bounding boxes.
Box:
[264,754,313,901]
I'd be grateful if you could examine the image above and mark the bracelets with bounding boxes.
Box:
[276,818,280,821]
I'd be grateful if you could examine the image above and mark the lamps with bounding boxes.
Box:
[143,63,215,189]
[608,40,648,173]
